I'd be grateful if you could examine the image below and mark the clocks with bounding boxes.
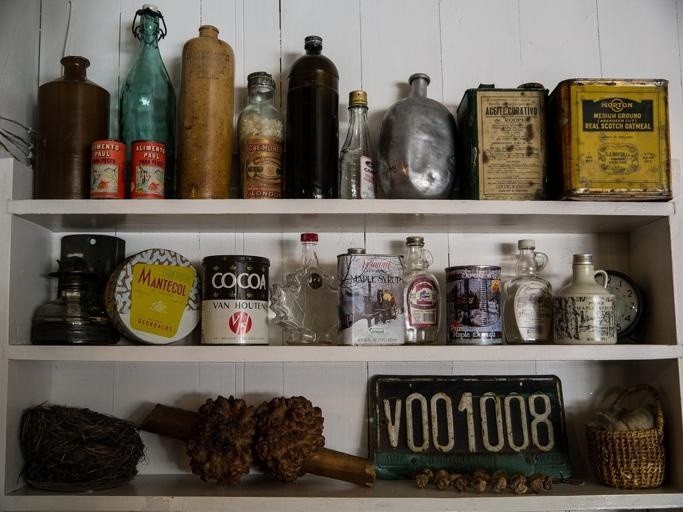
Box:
[586,268,643,339]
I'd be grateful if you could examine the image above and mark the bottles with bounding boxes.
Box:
[238,71,285,199]
[29,255,122,347]
[284,35,338,200]
[117,4,178,200]
[550,252,617,345]
[337,90,378,199]
[504,238,551,343]
[380,73,457,199]
[175,25,233,200]
[31,56,109,200]
[269,232,338,344]
[402,235,440,344]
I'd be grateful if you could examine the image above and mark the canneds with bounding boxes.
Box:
[131,140,166,200]
[91,138,126,199]
[336,253,405,345]
[201,255,271,345]
[445,264,505,346]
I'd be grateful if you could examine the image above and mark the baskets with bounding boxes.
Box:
[585,384,665,489]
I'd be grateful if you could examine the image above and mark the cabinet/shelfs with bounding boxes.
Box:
[3,195,681,512]
